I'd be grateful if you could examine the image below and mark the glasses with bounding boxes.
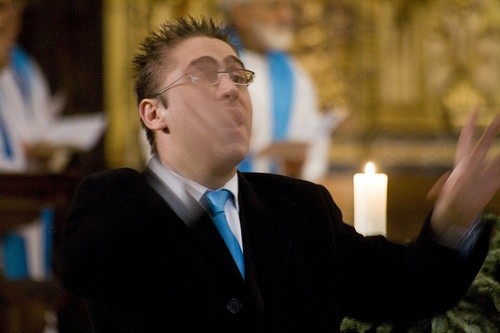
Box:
[152,69,255,97]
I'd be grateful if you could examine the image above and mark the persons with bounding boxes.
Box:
[56,16,500,333]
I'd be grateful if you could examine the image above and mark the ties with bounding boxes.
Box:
[200,191,244,279]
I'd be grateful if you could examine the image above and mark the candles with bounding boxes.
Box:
[352,163,389,240]
